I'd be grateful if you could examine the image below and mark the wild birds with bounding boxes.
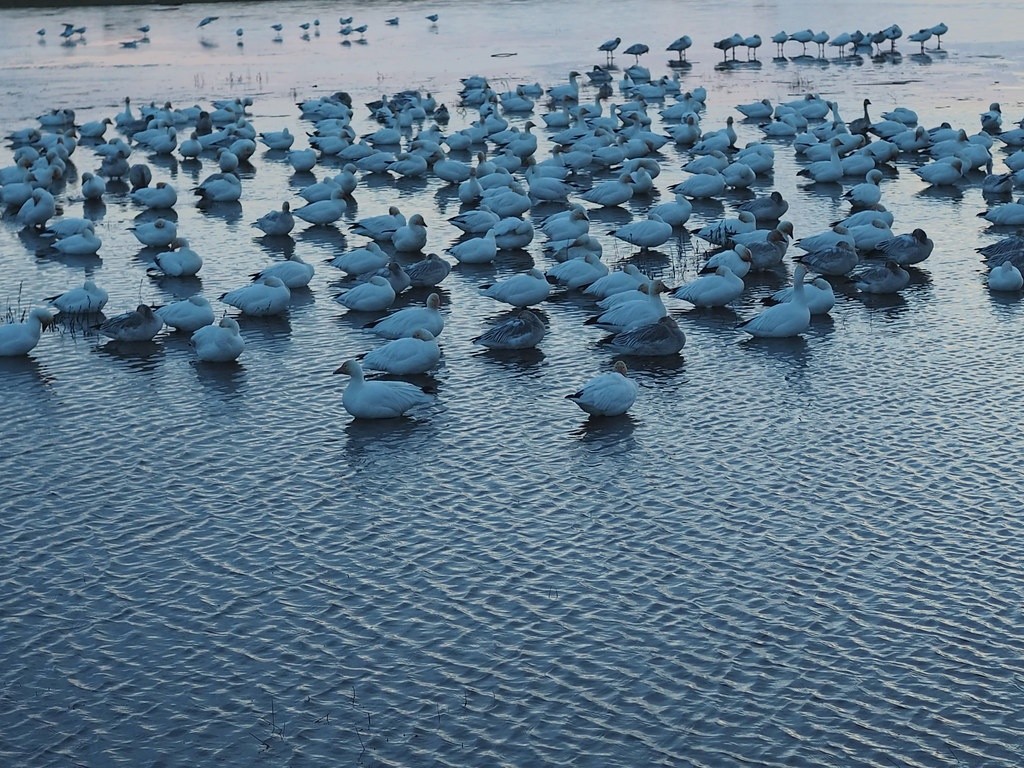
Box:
[298,22,311,30]
[565,360,639,417]
[384,17,399,24]
[271,23,282,35]
[469,310,546,349]
[594,316,686,356]
[235,28,243,37]
[597,37,621,59]
[361,328,441,376]
[0,307,54,357]
[769,23,902,59]
[195,17,219,29]
[666,35,692,60]
[58,23,87,41]
[333,360,435,419]
[623,44,649,62]
[0,64,1024,342]
[190,317,245,362]
[314,19,320,26]
[137,25,150,36]
[928,23,948,43]
[714,33,762,63]
[338,16,368,35]
[37,28,46,38]
[425,14,439,23]
[908,29,933,48]
